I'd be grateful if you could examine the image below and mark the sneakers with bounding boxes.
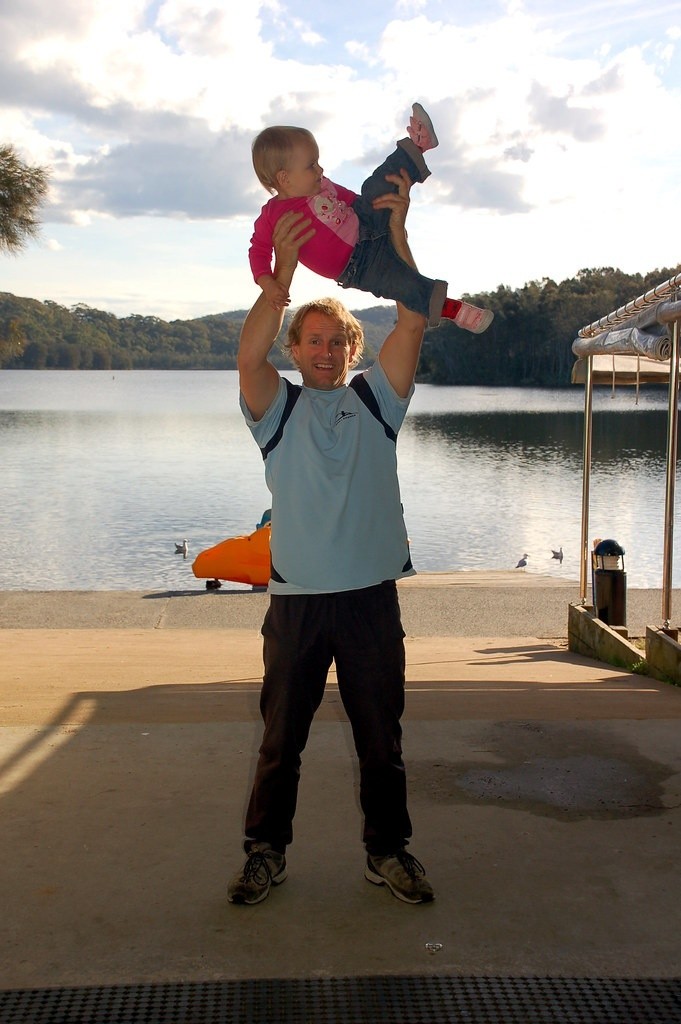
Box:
[364,845,436,905]
[451,301,495,333]
[226,838,288,905]
[407,102,439,155]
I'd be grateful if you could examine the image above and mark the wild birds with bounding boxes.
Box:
[516,552,529,572]
[550,548,566,557]
[174,539,188,552]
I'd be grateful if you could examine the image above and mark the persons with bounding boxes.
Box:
[248,103,493,333]
[227,168,436,905]
